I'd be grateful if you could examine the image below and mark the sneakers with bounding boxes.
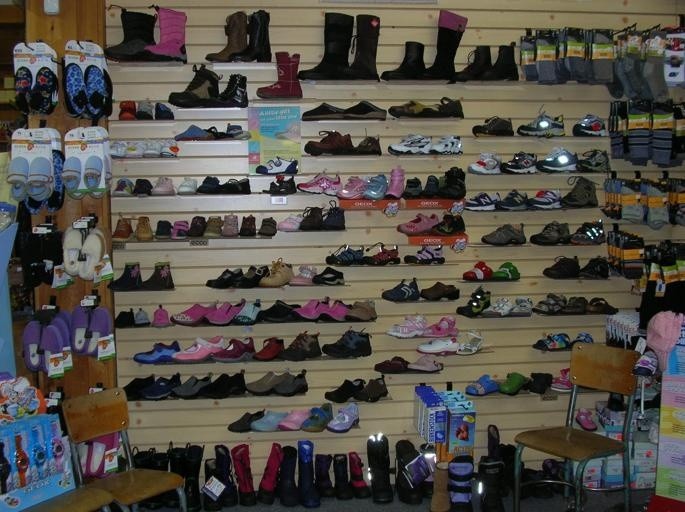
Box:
[4,61,116,481]
[115,57,630,436]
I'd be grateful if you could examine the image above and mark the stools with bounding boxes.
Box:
[21,490,114,510]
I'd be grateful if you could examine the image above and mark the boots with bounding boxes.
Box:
[454,42,495,80]
[295,11,355,81]
[480,43,527,81]
[204,11,250,64]
[255,50,303,100]
[382,40,428,81]
[416,7,469,81]
[102,4,158,64]
[226,10,274,65]
[341,13,381,82]
[142,6,190,66]
[127,422,591,512]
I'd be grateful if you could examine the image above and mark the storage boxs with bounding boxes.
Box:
[572,312,685,511]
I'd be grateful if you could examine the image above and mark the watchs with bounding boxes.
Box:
[32,426,48,481]
[14,434,30,488]
[50,421,64,473]
[0,441,11,494]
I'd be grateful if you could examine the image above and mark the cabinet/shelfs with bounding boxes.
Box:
[110,60,608,444]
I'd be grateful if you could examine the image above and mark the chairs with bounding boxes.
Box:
[64,388,189,511]
[517,333,638,512]
